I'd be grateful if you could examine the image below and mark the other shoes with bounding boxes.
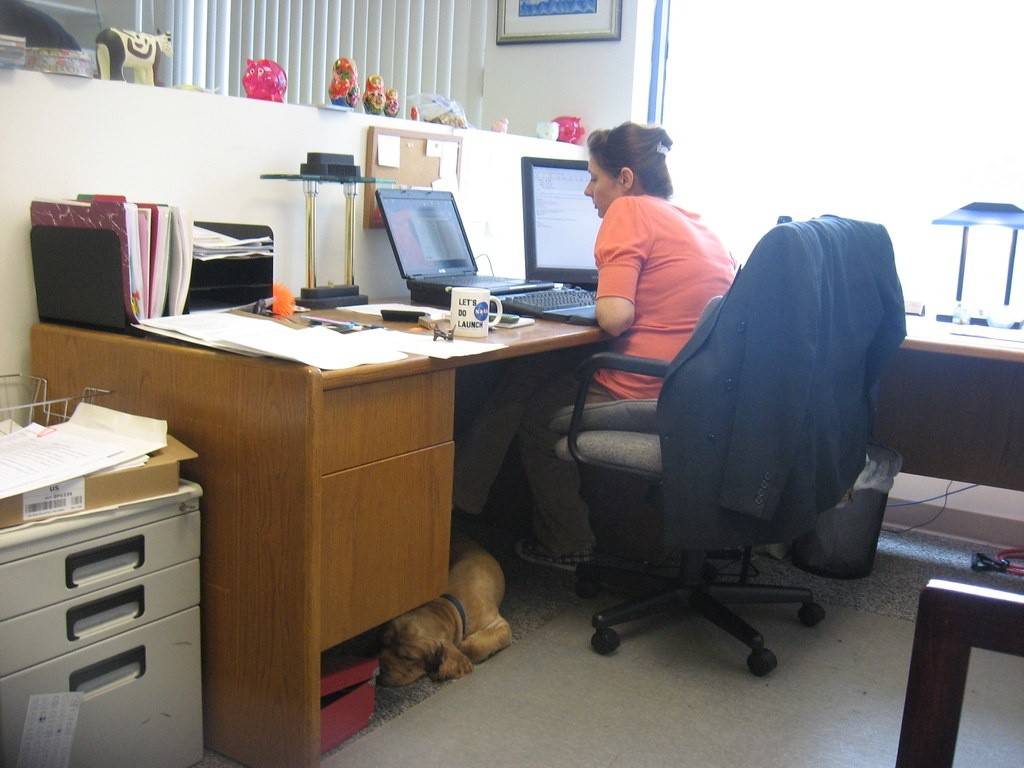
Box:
[514,537,592,571]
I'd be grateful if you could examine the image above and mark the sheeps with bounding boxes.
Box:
[492,119,509,134]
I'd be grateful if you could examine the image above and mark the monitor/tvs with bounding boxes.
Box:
[521,157,604,291]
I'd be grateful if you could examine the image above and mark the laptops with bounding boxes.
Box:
[375,189,555,297]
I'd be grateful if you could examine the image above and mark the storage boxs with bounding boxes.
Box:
[318,655,382,753]
[0,434,200,530]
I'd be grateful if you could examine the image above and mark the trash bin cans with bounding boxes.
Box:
[793,431,903,585]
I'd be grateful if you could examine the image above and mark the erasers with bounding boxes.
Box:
[417,316,435,330]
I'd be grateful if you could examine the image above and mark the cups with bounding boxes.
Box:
[450,288,503,337]
[537,122,559,140]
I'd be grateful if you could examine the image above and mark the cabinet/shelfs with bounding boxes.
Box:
[0,480,204,768]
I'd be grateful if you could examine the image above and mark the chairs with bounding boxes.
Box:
[569,219,895,677]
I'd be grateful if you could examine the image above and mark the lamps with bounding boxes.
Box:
[262,154,393,309]
[932,201,1024,330]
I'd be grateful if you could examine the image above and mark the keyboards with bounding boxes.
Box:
[503,289,596,313]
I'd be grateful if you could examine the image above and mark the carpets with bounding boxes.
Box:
[301,577,1024,768]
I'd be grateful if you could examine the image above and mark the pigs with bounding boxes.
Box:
[552,117,585,144]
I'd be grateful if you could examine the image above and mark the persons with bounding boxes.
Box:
[451,121,735,570]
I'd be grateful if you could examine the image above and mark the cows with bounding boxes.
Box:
[95,28,174,87]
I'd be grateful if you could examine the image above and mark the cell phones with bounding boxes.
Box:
[488,315,520,324]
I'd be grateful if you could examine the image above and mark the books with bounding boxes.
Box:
[31,194,195,323]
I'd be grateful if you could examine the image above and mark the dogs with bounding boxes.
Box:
[370,527,513,686]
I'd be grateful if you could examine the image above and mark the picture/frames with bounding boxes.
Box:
[497,0,624,44]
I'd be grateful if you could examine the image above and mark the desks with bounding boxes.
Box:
[29,278,615,768]
